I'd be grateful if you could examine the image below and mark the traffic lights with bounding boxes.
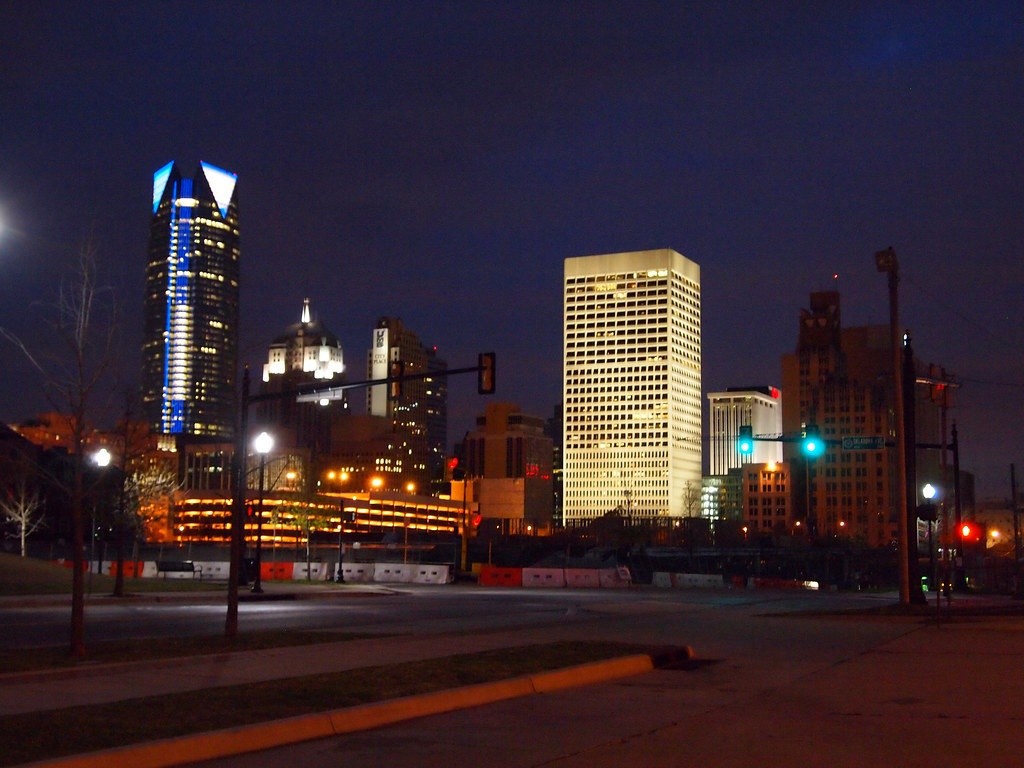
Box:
[805,424,822,465]
[471,512,482,529]
[443,454,459,481]
[737,424,754,455]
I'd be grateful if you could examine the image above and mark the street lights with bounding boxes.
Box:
[92,447,113,577]
[872,243,913,606]
[249,428,275,595]
[923,483,936,582]
